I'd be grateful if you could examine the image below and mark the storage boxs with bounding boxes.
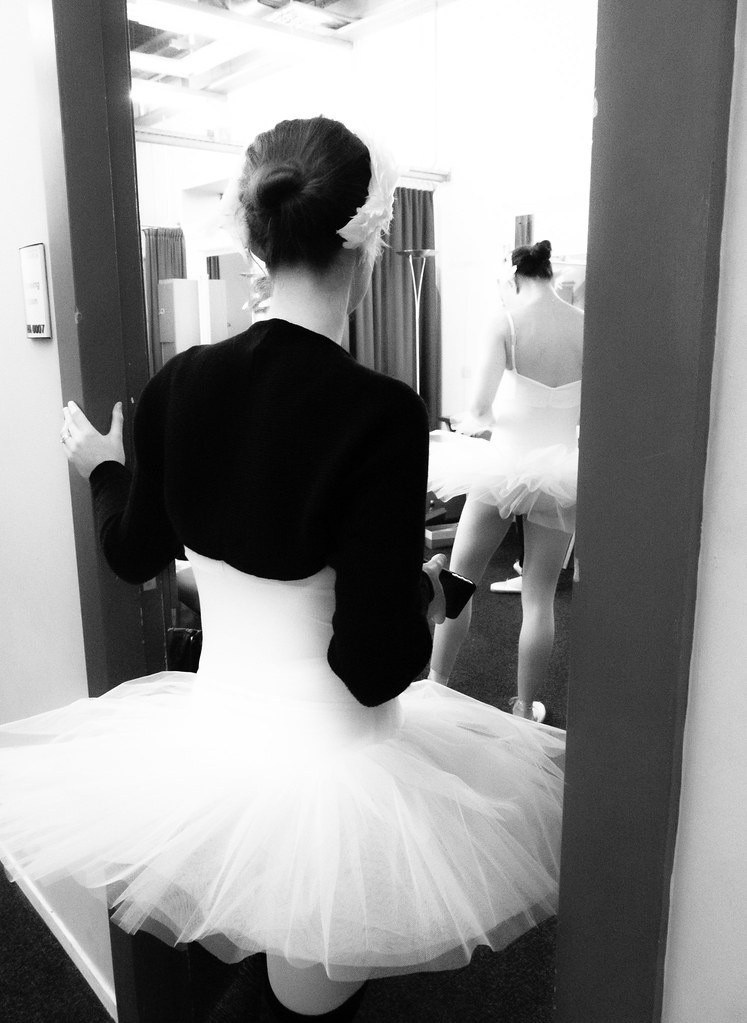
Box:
[425,521,458,549]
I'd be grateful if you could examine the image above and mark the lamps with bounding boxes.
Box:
[397,249,437,395]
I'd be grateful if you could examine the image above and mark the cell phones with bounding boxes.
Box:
[423,558,476,618]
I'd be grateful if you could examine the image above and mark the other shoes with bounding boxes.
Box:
[490,576,522,593]
[531,702,546,721]
[514,561,523,574]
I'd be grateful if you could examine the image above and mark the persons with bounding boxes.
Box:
[246,239,583,729]
[1,115,566,1023]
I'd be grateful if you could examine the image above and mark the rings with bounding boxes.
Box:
[61,432,70,444]
